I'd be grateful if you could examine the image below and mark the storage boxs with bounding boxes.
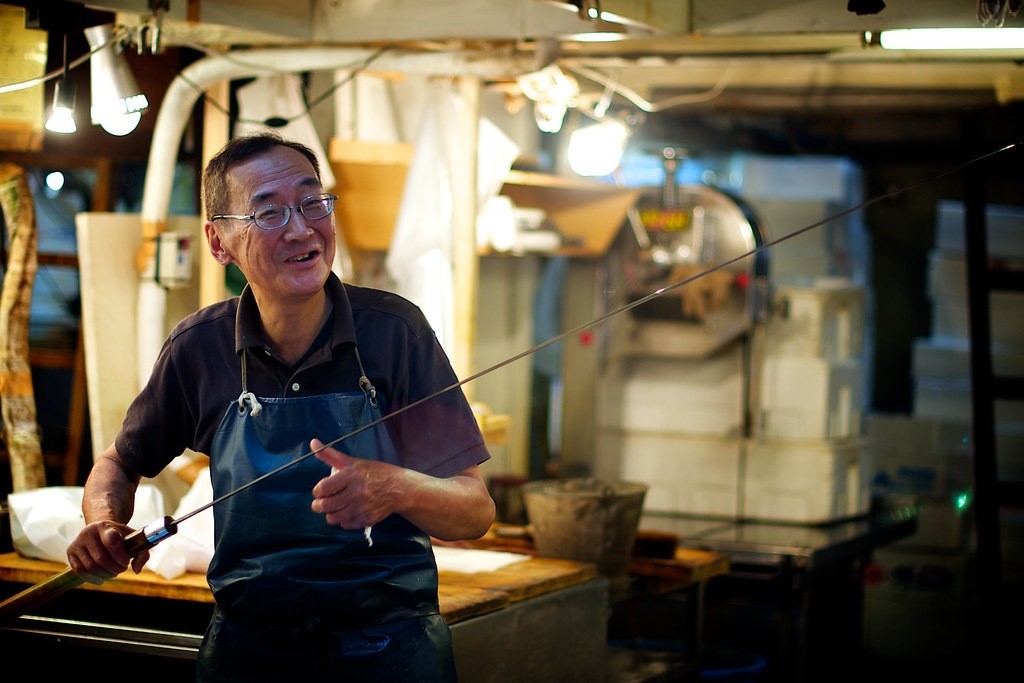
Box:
[745,361,869,442]
[744,439,871,523]
[750,285,864,360]
[595,340,748,438]
[592,431,742,518]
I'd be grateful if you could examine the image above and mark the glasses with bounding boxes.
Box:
[212,192,338,229]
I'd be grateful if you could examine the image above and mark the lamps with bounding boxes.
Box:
[862,28,1024,50]
[45,36,77,133]
[84,23,149,137]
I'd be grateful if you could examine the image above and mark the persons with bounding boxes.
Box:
[66,130,499,683]
